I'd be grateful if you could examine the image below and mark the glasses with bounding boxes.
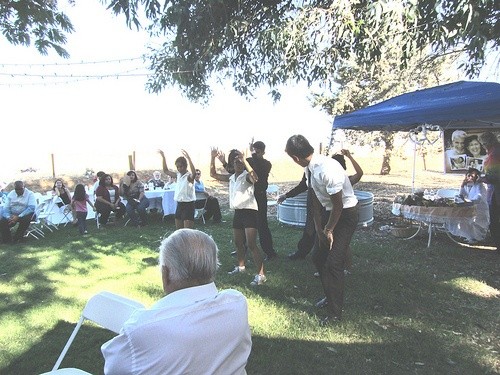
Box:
[196,173,200,175]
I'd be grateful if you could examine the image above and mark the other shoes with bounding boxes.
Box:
[316,296,328,308]
[464,238,478,243]
[230,250,238,255]
[287,251,296,257]
[314,272,320,277]
[319,313,338,327]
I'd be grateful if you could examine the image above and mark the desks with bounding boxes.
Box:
[144,189,177,224]
[40,191,96,225]
[392,201,479,248]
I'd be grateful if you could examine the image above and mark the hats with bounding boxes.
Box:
[127,170,135,176]
[468,167,481,177]
[332,155,345,170]
[228,150,243,164]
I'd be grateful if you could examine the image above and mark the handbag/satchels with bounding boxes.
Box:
[194,208,206,224]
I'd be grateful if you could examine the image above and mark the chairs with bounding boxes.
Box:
[195,192,208,225]
[25,195,132,241]
[38,289,147,375]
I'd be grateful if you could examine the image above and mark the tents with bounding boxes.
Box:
[323,80,500,196]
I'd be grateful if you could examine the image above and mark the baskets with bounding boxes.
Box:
[390,219,419,238]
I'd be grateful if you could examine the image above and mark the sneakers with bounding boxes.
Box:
[250,274,267,285]
[228,265,246,274]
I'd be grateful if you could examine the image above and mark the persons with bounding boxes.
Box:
[459,167,486,202]
[53,179,78,224]
[474,131,500,234]
[71,184,96,234]
[0,181,37,242]
[157,148,196,231]
[91,171,148,228]
[216,137,277,261]
[194,169,222,223]
[101,228,252,375]
[208,146,267,285]
[276,137,364,317]
[447,130,484,170]
[145,169,165,190]
